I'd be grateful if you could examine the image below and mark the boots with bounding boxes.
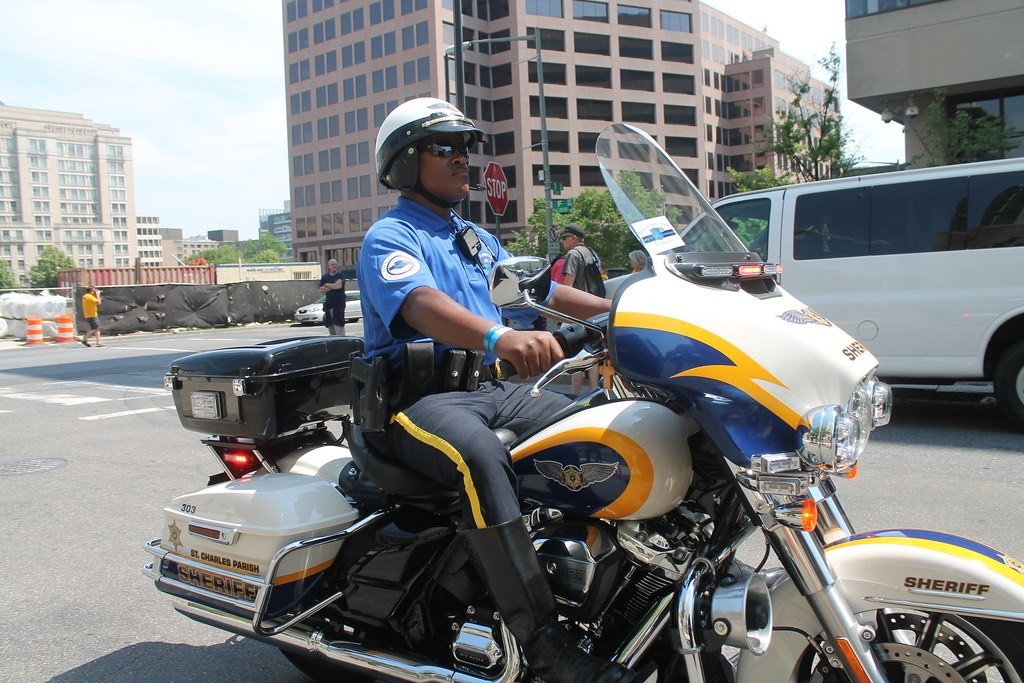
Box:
[465,512,633,682]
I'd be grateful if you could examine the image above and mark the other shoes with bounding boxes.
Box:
[96,344,103,346]
[82,339,90,346]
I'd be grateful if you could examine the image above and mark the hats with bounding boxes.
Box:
[555,223,586,238]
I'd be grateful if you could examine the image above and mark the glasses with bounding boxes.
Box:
[418,140,469,158]
[562,235,573,240]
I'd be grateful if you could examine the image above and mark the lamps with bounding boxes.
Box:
[881,99,894,124]
[905,94,920,119]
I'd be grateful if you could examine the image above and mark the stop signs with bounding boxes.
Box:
[483,163,509,217]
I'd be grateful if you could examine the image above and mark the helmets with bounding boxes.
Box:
[373,96,489,191]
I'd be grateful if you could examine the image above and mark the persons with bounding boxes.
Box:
[628,250,646,273]
[556,225,606,396]
[319,259,347,336]
[82,286,105,347]
[356,98,643,683]
[545,252,567,331]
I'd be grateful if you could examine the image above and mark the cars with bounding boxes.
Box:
[295,289,362,324]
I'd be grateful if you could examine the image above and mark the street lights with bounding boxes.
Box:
[445,34,558,263]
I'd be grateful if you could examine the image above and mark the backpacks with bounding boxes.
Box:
[573,245,607,297]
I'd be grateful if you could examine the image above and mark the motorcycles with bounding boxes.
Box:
[144,121,1024,683]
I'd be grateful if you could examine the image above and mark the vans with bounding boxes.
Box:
[603,155,1024,418]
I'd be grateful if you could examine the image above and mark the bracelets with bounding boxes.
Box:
[484,324,507,351]
[489,327,514,352]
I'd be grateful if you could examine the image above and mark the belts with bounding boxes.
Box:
[397,365,496,384]
